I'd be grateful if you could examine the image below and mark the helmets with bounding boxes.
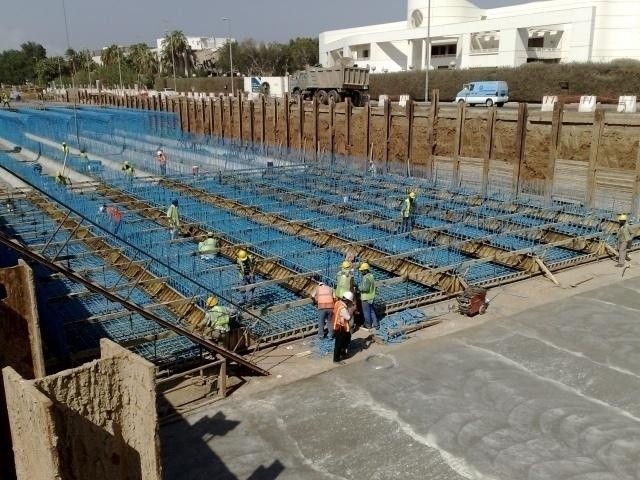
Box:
[208,232,213,237]
[342,261,352,270]
[238,251,248,261]
[619,215,627,221]
[410,192,416,199]
[343,291,354,302]
[207,297,219,307]
[359,263,369,270]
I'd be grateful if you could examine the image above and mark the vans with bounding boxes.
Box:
[455,80,509,107]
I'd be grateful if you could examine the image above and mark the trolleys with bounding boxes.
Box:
[458,285,500,316]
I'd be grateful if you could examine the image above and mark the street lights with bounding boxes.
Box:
[221,17,234,96]
[32,56,37,63]
[165,29,177,92]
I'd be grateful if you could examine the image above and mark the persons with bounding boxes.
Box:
[311,282,335,339]
[99,205,122,235]
[368,161,378,178]
[400,192,416,243]
[80,149,89,173]
[197,230,218,259]
[156,150,166,174]
[166,198,180,244]
[197,296,239,342]
[335,260,356,309]
[62,142,67,154]
[236,250,258,308]
[2,92,10,107]
[332,291,355,363]
[55,171,66,185]
[121,161,136,177]
[353,262,380,330]
[615,215,634,266]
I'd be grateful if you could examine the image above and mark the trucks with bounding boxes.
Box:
[290,64,370,107]
